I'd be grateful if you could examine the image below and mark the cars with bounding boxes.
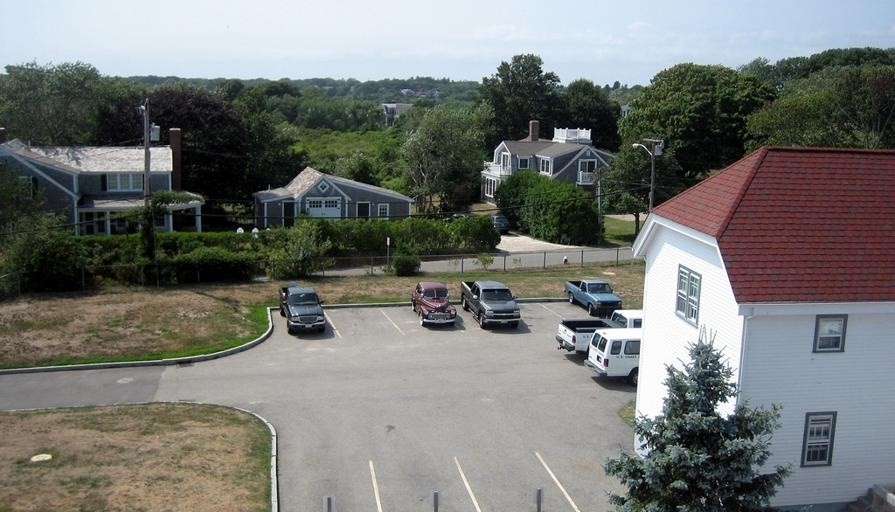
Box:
[492,215,510,235]
[411,281,457,326]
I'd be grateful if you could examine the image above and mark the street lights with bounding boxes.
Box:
[633,135,664,209]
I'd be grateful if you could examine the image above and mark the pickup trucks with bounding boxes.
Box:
[280,287,325,334]
[462,280,522,329]
[556,279,642,387]
